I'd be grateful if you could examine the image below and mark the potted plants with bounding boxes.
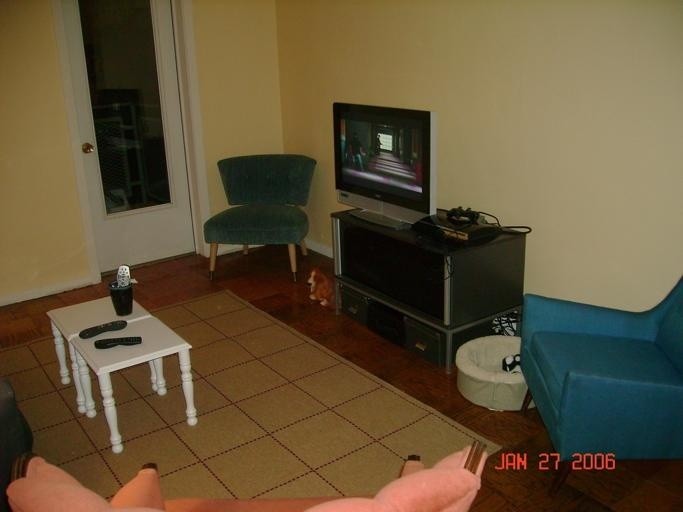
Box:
[109,264,138,315]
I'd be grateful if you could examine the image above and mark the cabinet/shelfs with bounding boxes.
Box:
[329,208,526,378]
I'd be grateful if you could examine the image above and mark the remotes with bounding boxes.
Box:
[94,337,142,350]
[78,320,127,339]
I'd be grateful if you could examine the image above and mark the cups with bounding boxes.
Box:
[108,281,134,316]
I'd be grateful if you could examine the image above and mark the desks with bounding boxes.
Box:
[45,292,199,455]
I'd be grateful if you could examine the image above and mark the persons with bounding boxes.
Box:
[350,131,364,172]
[375,134,382,154]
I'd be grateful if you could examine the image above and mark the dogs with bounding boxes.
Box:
[306,268,336,311]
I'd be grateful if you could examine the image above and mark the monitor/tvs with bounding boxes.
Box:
[330,99,438,230]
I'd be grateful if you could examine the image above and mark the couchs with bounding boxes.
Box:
[7,443,487,512]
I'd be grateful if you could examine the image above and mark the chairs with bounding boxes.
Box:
[520,274,683,498]
[203,154,318,282]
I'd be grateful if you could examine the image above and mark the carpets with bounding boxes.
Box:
[1,289,504,499]
